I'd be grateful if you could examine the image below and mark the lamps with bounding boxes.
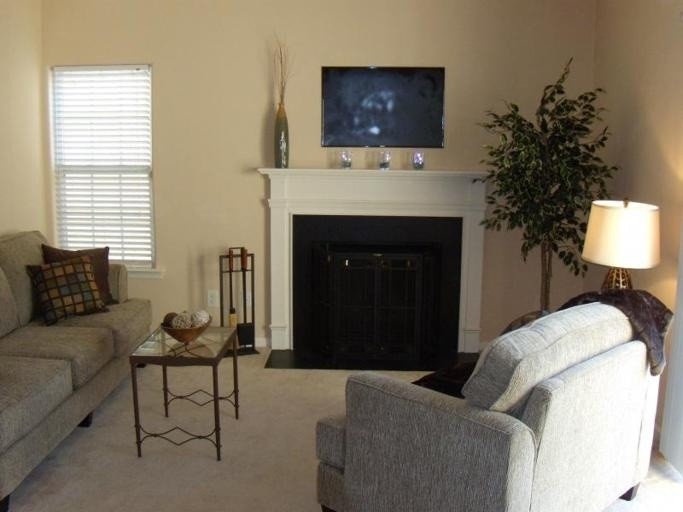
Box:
[580,198,660,294]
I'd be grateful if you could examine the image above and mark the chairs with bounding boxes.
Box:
[315,302,663,511]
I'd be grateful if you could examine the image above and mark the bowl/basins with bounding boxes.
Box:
[160,316,213,343]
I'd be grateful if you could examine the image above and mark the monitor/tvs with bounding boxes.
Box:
[320,65,445,149]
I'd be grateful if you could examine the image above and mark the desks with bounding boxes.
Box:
[130,324,239,461]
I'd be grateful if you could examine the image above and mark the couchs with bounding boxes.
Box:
[0,231,153,512]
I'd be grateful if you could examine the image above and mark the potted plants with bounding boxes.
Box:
[275,36,287,167]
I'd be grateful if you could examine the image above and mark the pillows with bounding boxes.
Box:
[0,230,119,338]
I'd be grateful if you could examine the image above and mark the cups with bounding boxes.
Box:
[339,151,353,170]
[377,150,391,170]
[413,151,425,169]
